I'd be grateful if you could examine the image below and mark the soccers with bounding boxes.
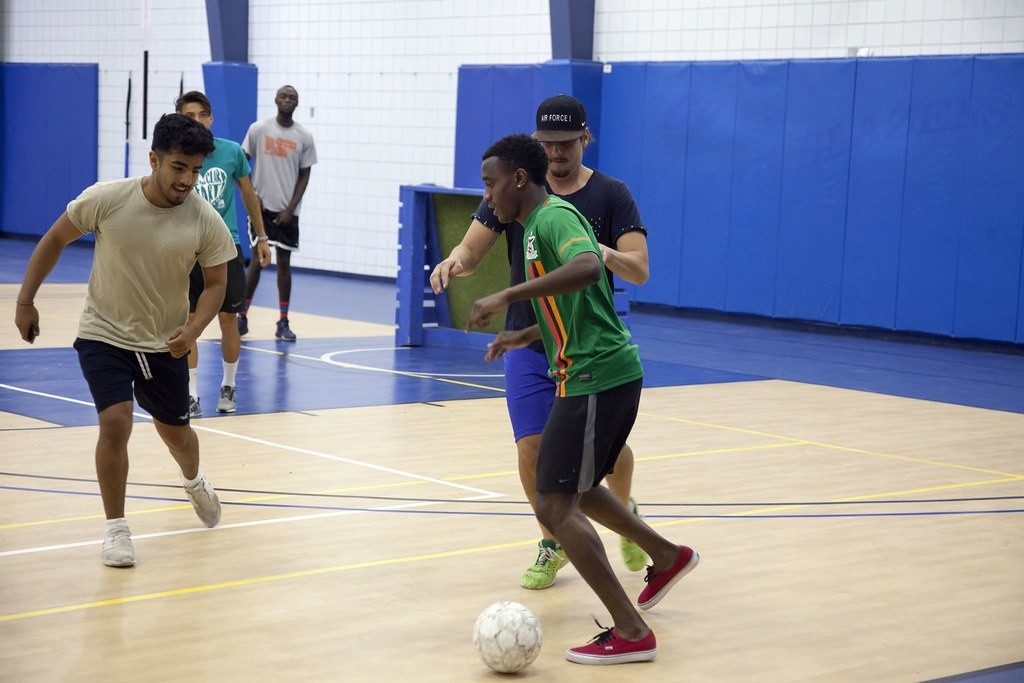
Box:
[469,598,545,675]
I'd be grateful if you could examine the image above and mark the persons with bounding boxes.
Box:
[236,85,320,342]
[15,113,239,566]
[173,91,272,418]
[429,94,650,589]
[464,133,700,666]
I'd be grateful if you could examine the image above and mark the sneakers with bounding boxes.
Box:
[521,539,570,590]
[184,476,221,529]
[619,497,647,572]
[566,618,656,665]
[102,526,136,567]
[275,317,296,342]
[216,384,236,414]
[187,396,203,417]
[237,316,248,335]
[637,545,699,610]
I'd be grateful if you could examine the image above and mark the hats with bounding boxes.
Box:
[530,94,588,142]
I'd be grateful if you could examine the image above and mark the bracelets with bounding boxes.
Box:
[258,235,268,242]
[17,299,34,306]
